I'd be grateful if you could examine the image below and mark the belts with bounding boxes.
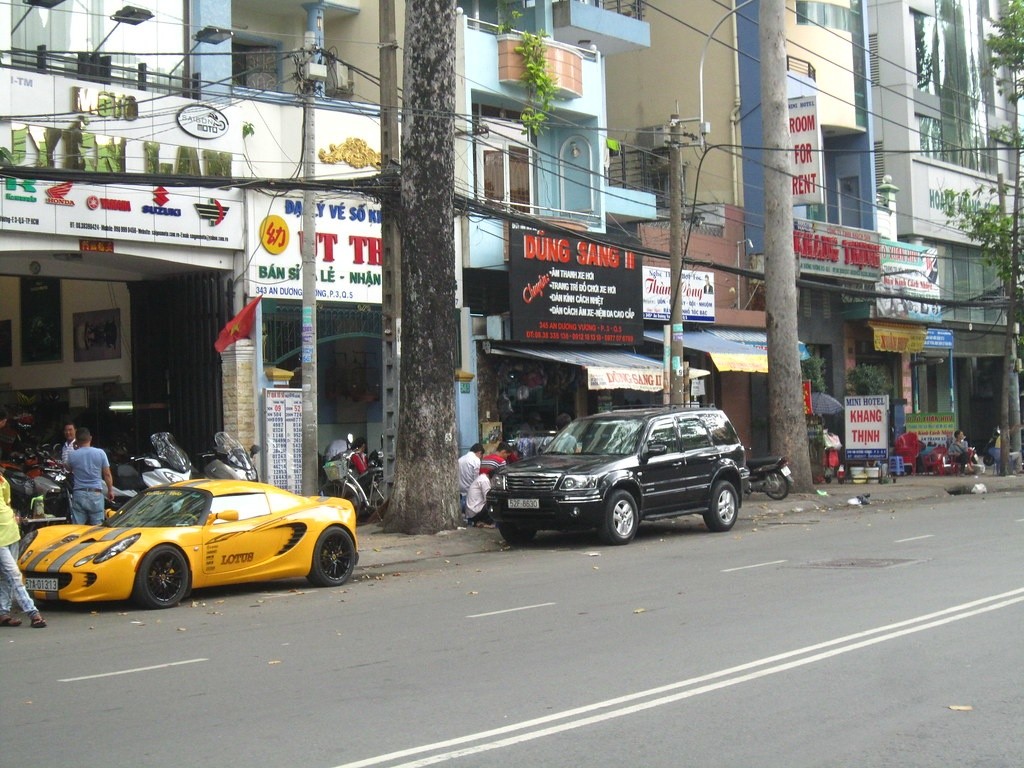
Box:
[79,487,101,492]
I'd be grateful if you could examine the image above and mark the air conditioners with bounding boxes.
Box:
[636,125,671,148]
[325,60,348,95]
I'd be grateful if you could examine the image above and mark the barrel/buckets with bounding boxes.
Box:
[865,467,879,483]
[850,466,864,476]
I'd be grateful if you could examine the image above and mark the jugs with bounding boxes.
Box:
[30,495,44,519]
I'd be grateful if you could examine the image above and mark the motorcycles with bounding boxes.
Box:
[742,446,794,501]
[186,431,261,483]
[1,430,111,522]
[104,431,197,511]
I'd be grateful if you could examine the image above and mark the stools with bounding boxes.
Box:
[890,456,905,479]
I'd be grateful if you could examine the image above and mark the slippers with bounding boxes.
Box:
[30,617,48,627]
[0,617,22,626]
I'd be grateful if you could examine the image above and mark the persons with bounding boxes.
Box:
[951,430,977,465]
[61,422,77,473]
[554,412,577,453]
[66,427,114,526]
[0,475,47,628]
[323,437,369,478]
[704,275,713,294]
[458,441,519,526]
[0,408,8,428]
[996,428,1024,474]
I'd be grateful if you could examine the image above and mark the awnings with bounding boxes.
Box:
[496,344,710,392]
[869,323,927,353]
[642,330,812,373]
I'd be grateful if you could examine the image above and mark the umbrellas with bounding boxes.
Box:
[811,391,844,416]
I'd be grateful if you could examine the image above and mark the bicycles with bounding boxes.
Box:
[320,431,395,524]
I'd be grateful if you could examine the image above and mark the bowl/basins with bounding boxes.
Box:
[853,475,868,482]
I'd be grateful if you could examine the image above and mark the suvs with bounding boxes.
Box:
[486,404,751,546]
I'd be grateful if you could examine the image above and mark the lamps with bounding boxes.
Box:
[168,26,234,95]
[882,175,892,184]
[90,6,155,58]
[11,0,66,35]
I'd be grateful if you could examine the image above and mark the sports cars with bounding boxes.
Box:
[20,478,361,611]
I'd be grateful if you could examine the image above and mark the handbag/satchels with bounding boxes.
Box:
[822,429,842,451]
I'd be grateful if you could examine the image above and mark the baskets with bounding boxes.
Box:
[323,461,348,480]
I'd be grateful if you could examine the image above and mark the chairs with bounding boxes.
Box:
[925,447,974,476]
[892,431,919,475]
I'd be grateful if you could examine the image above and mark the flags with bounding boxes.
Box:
[215,295,262,352]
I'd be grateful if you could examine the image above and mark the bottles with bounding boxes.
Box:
[838,464,844,485]
